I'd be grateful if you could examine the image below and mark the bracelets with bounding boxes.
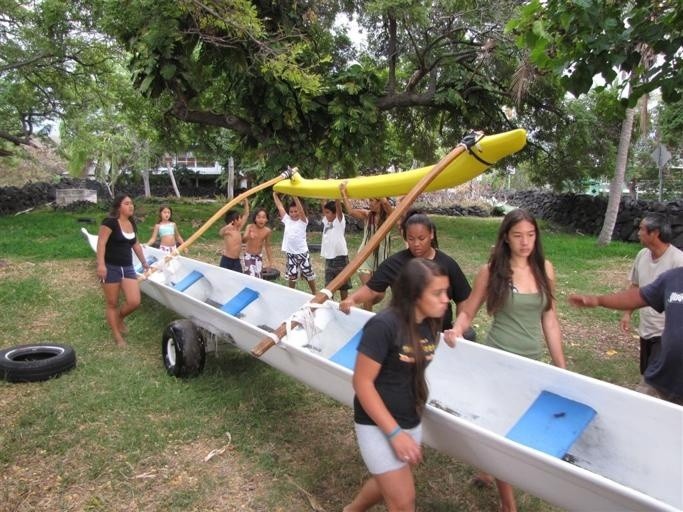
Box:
[385,426,402,440]
[140,259,146,265]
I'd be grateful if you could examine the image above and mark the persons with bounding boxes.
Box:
[142,205,188,258]
[317,196,352,305]
[617,211,682,378]
[338,180,394,312]
[269,191,316,295]
[339,257,450,512]
[95,190,150,346]
[441,206,567,512]
[241,207,272,280]
[337,212,474,338]
[566,265,682,408]
[217,197,249,272]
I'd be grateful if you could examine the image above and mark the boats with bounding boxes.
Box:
[81,225,682,511]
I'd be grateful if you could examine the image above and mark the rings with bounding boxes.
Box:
[401,455,410,461]
